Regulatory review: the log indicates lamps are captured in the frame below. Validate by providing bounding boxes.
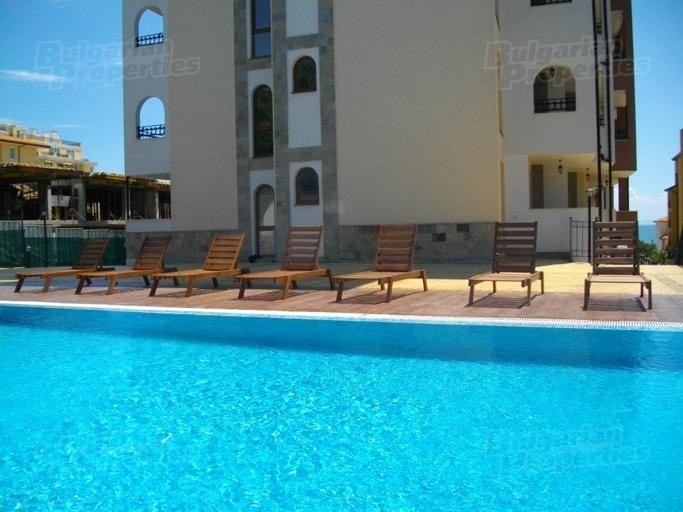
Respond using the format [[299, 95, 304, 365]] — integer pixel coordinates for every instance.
[[557, 160, 609, 187]]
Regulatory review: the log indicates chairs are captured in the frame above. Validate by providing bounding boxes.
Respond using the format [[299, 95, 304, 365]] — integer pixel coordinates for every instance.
[[149, 232, 251, 298], [469, 222, 543, 305], [233, 224, 334, 299], [583, 221, 651, 311], [74, 235, 180, 295], [333, 224, 428, 304], [14, 239, 115, 292]]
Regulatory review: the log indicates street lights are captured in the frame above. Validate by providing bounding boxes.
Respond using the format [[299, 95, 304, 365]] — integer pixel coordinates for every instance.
[[584, 186, 594, 263], [40, 207, 47, 267]]
